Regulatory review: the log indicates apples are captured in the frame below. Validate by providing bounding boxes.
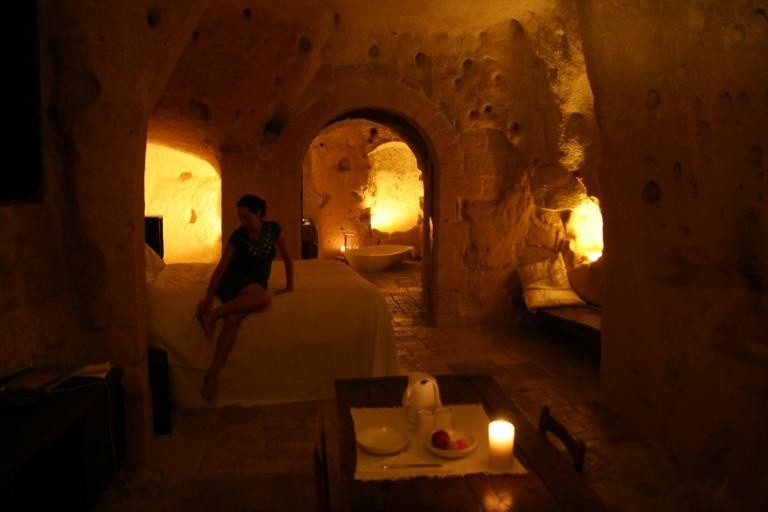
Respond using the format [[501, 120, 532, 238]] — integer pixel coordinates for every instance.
[[431, 430, 450, 449]]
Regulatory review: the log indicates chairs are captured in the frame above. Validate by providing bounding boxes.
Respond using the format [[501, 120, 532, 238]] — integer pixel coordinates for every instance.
[[309, 405, 586, 511]]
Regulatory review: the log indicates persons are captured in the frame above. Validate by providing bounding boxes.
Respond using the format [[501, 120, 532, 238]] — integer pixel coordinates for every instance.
[[194, 193, 294, 401]]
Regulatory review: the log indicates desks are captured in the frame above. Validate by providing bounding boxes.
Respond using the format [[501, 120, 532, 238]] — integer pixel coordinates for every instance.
[[1, 365, 126, 511]]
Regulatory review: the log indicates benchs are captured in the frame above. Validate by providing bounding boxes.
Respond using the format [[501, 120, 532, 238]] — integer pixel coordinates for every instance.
[[512, 287, 601, 342]]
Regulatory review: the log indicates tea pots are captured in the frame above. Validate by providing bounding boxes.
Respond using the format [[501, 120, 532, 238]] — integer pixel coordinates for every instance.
[[403, 377, 440, 422]]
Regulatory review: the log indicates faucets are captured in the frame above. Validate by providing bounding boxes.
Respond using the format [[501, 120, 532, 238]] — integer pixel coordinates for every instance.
[[344, 233, 355, 250]]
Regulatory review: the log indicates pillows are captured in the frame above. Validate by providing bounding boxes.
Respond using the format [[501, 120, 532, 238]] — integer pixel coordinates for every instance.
[[514, 253, 587, 314]]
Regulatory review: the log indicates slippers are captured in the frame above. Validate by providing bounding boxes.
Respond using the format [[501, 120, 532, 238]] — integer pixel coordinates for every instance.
[[202, 372, 217, 400], [197, 313, 214, 340]]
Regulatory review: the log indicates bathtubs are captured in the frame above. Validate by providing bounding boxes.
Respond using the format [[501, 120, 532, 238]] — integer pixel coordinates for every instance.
[[345, 244, 409, 273]]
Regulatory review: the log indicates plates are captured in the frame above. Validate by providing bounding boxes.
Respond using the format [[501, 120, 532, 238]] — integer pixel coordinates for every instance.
[[355, 427, 410, 458], [424, 431, 478, 458]]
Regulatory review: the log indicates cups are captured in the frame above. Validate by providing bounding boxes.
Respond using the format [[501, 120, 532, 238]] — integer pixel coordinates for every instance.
[[416, 407, 451, 437]]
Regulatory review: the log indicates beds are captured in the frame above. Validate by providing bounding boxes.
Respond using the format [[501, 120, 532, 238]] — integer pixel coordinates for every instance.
[[145, 258, 393, 406]]
